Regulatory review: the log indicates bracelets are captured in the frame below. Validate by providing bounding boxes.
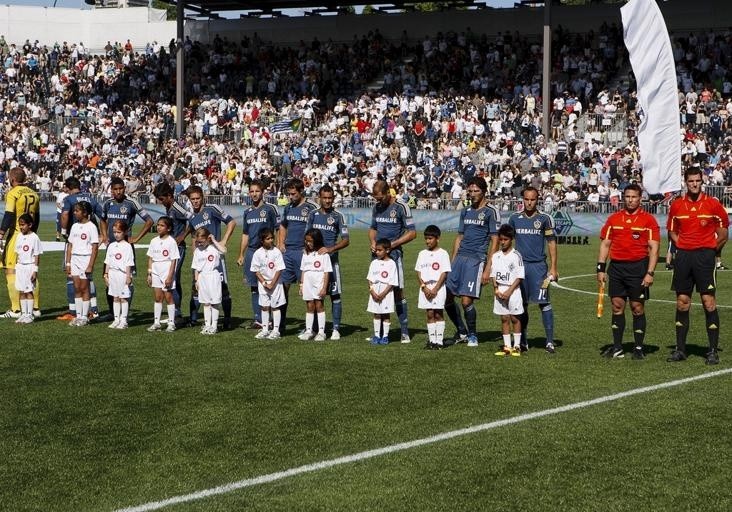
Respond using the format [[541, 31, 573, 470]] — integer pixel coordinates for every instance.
[[493, 285, 498, 289], [368, 285, 373, 289], [369, 287, 374, 291], [103, 275, 107, 278], [262, 281, 268, 287], [146, 272, 152, 277], [194, 279, 198, 285], [298, 281, 304, 284], [421, 283, 425, 288], [422, 286, 426, 290], [494, 289, 500, 294], [298, 283, 303, 287], [261, 281, 266, 286]]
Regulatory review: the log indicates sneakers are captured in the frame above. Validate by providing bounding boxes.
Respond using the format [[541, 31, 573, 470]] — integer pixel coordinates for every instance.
[[547, 342, 557, 352], [665, 264, 673, 270], [0, 309, 41, 323], [365, 333, 388, 344], [186, 317, 231, 334], [496, 345, 529, 357], [245, 322, 281, 339], [299, 328, 342, 341], [400, 332, 411, 344], [600, 347, 624, 357], [632, 345, 644, 359], [57, 311, 100, 325], [424, 333, 479, 350], [705, 348, 718, 365], [146, 316, 184, 332], [109, 318, 129, 329], [717, 263, 728, 269], [667, 349, 689, 361]]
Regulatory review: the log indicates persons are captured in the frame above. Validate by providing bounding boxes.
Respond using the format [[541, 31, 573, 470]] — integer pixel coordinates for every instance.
[[366, 237, 399, 345], [298, 227, 333, 342], [55, 175, 103, 323], [489, 223, 527, 358], [14, 212, 44, 324], [506, 186, 559, 354], [364, 180, 417, 345], [236, 178, 281, 331], [596, 184, 661, 363], [97, 176, 155, 322], [145, 215, 181, 332], [189, 227, 227, 335], [665, 166, 730, 365], [182, 184, 237, 330], [249, 227, 287, 341], [0, 167, 41, 319], [1, 16, 732, 274], [65, 200, 100, 327], [305, 184, 350, 341], [444, 175, 501, 348], [414, 224, 453, 351], [104, 220, 135, 330], [279, 177, 318, 336], [151, 182, 195, 324]]
